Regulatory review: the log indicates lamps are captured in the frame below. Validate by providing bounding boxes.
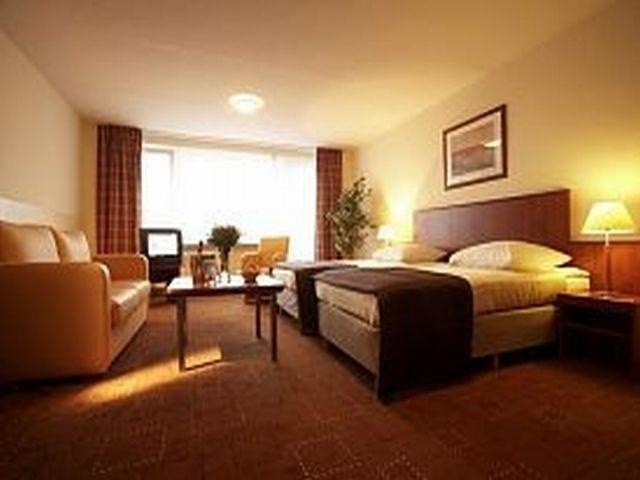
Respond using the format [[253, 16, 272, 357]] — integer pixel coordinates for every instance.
[[376, 223, 397, 247], [228, 93, 263, 113], [581, 201, 635, 293]]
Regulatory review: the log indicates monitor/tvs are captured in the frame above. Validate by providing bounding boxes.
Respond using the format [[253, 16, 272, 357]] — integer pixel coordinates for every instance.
[[146, 232, 179, 260]]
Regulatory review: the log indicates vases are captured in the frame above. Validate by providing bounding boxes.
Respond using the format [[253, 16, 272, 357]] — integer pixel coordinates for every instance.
[[219, 249, 230, 273]]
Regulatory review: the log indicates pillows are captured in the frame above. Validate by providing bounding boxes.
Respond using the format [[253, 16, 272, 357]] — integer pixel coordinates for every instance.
[[375, 242, 447, 265], [449, 238, 572, 273]]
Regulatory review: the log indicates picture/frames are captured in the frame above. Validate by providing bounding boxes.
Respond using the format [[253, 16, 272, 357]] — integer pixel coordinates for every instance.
[[444, 104, 508, 190]]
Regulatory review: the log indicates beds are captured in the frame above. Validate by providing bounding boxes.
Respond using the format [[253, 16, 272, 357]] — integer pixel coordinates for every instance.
[[310, 261, 593, 401], [270, 256, 398, 337]]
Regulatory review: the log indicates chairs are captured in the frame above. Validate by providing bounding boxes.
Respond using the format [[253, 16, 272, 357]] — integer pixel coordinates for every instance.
[[243, 235, 288, 277]]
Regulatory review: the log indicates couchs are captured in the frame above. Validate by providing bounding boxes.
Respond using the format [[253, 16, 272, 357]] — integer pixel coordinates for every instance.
[[0, 222, 151, 386]]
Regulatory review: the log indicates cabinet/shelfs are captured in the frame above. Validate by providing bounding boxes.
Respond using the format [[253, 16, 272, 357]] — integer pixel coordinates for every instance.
[[140, 229, 183, 282]]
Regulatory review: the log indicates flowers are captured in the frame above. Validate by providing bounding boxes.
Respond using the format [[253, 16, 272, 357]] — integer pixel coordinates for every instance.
[[208, 226, 242, 249]]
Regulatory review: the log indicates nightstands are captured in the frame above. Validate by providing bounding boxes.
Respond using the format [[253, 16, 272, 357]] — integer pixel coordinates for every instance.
[[554, 289, 640, 374]]
